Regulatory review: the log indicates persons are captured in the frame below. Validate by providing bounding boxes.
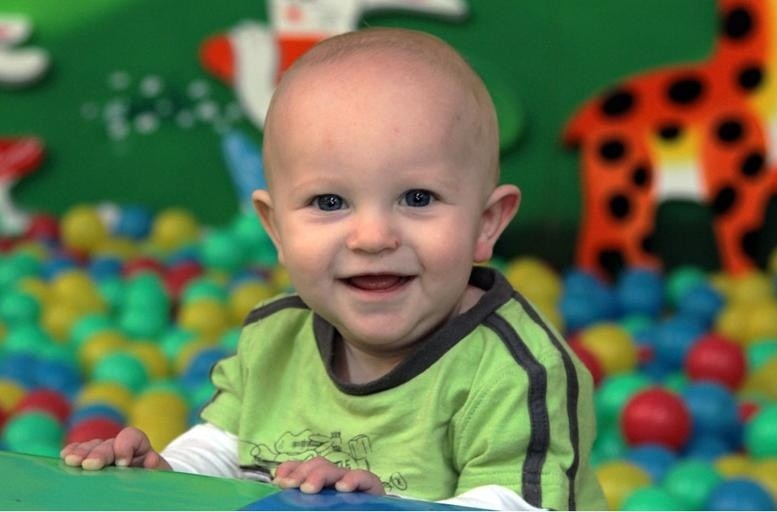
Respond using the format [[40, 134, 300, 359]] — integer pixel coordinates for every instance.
[[59, 24, 612, 512]]
